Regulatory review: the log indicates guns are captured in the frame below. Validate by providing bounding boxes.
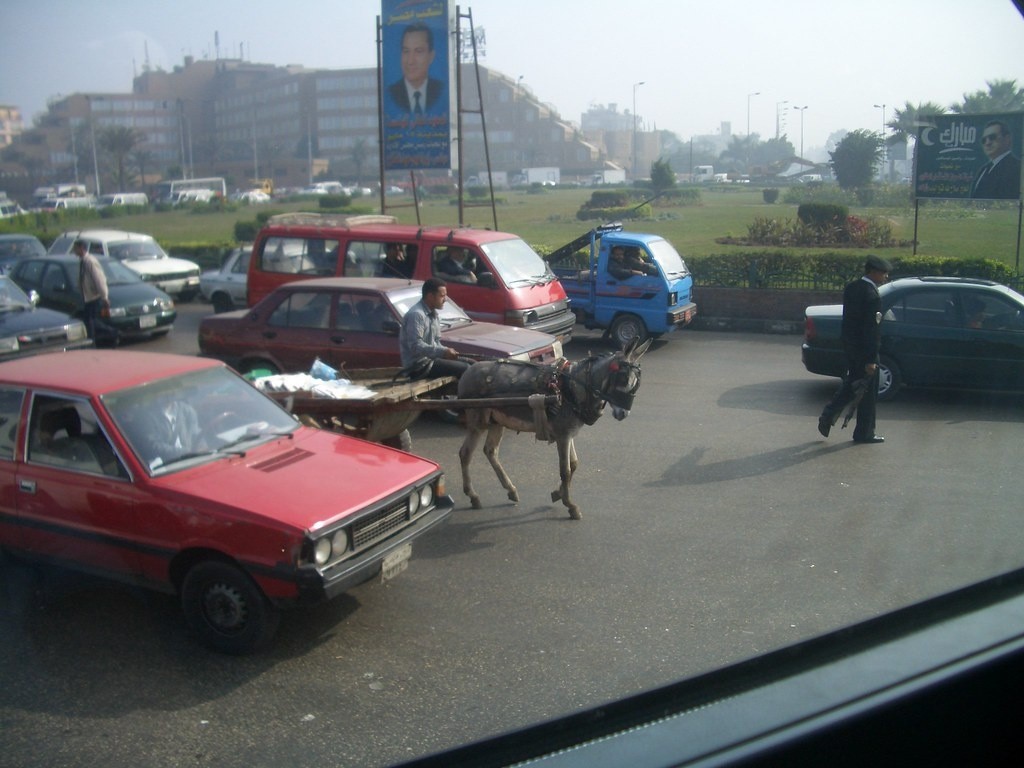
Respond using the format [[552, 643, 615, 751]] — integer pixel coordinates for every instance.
[[841, 363, 879, 430]]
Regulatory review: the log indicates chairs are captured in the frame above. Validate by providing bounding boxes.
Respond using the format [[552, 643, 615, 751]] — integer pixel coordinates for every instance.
[[34, 406, 121, 476], [335, 303, 355, 328], [356, 300, 385, 330]]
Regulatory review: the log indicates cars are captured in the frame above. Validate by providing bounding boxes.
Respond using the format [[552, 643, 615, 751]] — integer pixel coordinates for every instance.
[[0, 347, 458, 655], [198, 242, 334, 314], [0, 272, 95, 361], [674, 166, 821, 184], [0, 232, 48, 274], [45, 228, 202, 303], [197, 275, 567, 425], [800, 275, 1024, 405], [10, 252, 177, 349]]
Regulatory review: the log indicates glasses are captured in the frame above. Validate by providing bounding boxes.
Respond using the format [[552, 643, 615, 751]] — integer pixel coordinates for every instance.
[[981, 133, 996, 144]]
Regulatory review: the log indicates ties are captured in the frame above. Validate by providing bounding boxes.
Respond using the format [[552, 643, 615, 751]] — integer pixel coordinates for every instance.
[[982, 162, 994, 177], [412, 92, 422, 115]]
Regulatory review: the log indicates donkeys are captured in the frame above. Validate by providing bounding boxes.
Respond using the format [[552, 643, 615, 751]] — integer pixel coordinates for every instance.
[[456, 335, 653, 518]]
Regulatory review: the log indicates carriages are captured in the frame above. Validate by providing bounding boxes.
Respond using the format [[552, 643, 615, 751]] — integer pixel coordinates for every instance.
[[217, 332, 656, 522]]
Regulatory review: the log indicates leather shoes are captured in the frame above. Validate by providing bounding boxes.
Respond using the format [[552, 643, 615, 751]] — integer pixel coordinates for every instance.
[[854, 436, 884, 442], [818, 415, 831, 437]]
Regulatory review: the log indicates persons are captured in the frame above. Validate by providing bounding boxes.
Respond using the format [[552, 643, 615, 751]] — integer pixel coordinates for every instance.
[[75, 240, 122, 347], [376, 242, 418, 279], [398, 278, 478, 425], [959, 294, 1013, 330], [130, 388, 206, 463], [607, 246, 657, 280], [388, 22, 443, 114], [439, 245, 503, 286], [818, 253, 892, 441]]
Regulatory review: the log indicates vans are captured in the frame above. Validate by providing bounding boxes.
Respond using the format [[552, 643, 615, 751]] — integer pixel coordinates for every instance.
[[247, 212, 576, 346]]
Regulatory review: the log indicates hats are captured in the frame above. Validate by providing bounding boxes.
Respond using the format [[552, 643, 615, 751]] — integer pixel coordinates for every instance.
[[867, 254, 893, 273]]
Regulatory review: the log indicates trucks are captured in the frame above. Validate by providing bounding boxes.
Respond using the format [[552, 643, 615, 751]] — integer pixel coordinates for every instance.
[[543, 223, 699, 350], [0, 167, 626, 220]]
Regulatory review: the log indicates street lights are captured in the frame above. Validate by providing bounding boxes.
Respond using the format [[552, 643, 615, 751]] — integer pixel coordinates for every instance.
[[516, 74, 525, 172], [747, 92, 761, 165], [873, 103, 887, 186], [633, 81, 646, 180], [793, 105, 808, 171]]
